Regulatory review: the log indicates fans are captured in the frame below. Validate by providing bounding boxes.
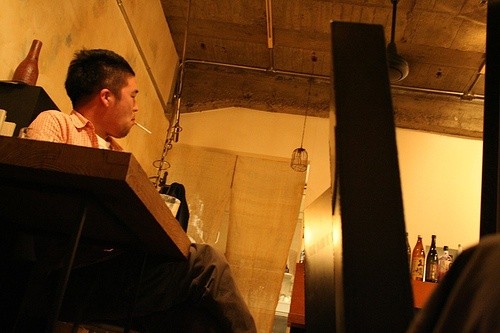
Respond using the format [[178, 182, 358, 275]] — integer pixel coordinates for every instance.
[[386, 0, 409, 83]]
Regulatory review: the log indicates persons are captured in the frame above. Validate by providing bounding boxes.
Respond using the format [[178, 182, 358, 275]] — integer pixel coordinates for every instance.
[[24, 48, 257, 333]]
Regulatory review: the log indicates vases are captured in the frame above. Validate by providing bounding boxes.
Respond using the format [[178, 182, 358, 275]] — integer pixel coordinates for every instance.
[[13, 40, 43, 85]]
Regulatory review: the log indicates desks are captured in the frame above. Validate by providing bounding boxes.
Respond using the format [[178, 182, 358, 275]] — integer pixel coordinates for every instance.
[[288, 253, 308, 325], [0, 134, 190, 333]]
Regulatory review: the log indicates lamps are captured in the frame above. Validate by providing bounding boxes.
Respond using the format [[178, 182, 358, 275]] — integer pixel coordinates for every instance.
[[291, 56, 318, 171]]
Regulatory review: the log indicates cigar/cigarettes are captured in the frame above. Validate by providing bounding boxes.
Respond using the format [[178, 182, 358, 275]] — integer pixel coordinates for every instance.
[[134, 121, 152, 135]]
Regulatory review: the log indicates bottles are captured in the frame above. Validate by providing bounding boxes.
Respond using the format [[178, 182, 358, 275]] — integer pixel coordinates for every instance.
[[12, 39, 42, 86], [457, 243, 462, 254], [405, 232, 411, 267], [426, 235, 438, 282], [438, 246, 452, 282], [412, 234, 426, 282]]
